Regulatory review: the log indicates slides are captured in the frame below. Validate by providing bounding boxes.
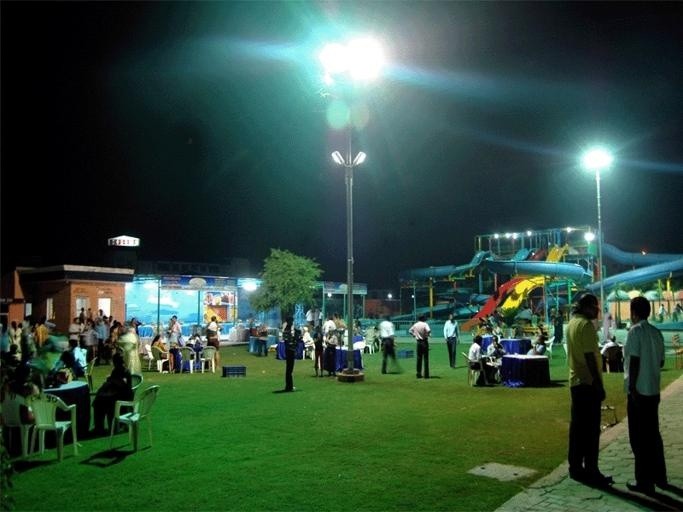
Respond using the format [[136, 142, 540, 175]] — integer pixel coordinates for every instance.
[[459, 246, 566, 332]]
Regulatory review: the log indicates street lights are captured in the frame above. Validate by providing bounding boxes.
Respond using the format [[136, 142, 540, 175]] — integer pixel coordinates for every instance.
[[318, 34, 389, 382], [578, 142, 617, 345]]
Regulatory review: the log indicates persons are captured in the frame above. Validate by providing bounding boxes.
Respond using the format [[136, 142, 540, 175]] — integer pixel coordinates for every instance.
[[468, 334, 493, 385], [88, 352, 134, 435], [274, 305, 381, 377], [407, 314, 432, 379], [565, 291, 614, 483], [378, 314, 399, 375], [258, 323, 268, 357], [622, 294, 672, 496], [283, 317, 301, 393], [443, 313, 461, 369], [487, 335, 506, 355], [658, 304, 683, 322], [8, 364, 41, 424], [600, 307, 620, 357], [526, 334, 545, 355], [0, 305, 239, 375], [554, 311, 564, 344]]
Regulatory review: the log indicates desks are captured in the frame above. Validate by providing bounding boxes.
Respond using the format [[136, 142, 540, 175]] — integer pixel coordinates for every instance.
[[475, 333, 503, 352], [501, 352, 551, 390], [0, 380, 98, 445], [498, 337, 534, 355]]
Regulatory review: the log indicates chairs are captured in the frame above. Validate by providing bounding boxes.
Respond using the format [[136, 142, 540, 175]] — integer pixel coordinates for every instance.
[[670, 331, 682, 374], [604, 346, 626, 374], [461, 350, 494, 388], [541, 346, 547, 356], [596, 400, 618, 426], [542, 336, 556, 355], [25, 390, 82, 462], [107, 384, 160, 455], [0, 386, 37, 462]]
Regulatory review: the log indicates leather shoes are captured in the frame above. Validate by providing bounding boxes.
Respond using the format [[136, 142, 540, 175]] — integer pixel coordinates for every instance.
[[588, 474, 612, 484], [627, 479, 654, 492]]
